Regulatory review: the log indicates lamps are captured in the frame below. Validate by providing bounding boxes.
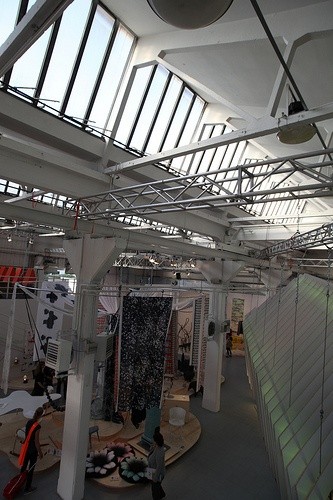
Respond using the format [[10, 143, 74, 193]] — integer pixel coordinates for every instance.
[[277, 99, 316, 145], [145, 0, 234, 31]]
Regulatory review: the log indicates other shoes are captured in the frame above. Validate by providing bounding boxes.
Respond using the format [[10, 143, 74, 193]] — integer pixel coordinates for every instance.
[[24, 487, 37, 496], [230, 355, 232, 357], [226, 356, 229, 358]]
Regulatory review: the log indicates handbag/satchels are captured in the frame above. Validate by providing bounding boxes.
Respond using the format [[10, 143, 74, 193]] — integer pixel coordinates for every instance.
[[18, 444, 28, 466]]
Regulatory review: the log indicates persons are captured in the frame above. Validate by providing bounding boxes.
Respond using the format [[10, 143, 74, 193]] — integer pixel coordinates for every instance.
[[20, 407, 43, 491], [146, 432, 166, 500], [31, 360, 48, 396], [226, 333, 232, 358]]
